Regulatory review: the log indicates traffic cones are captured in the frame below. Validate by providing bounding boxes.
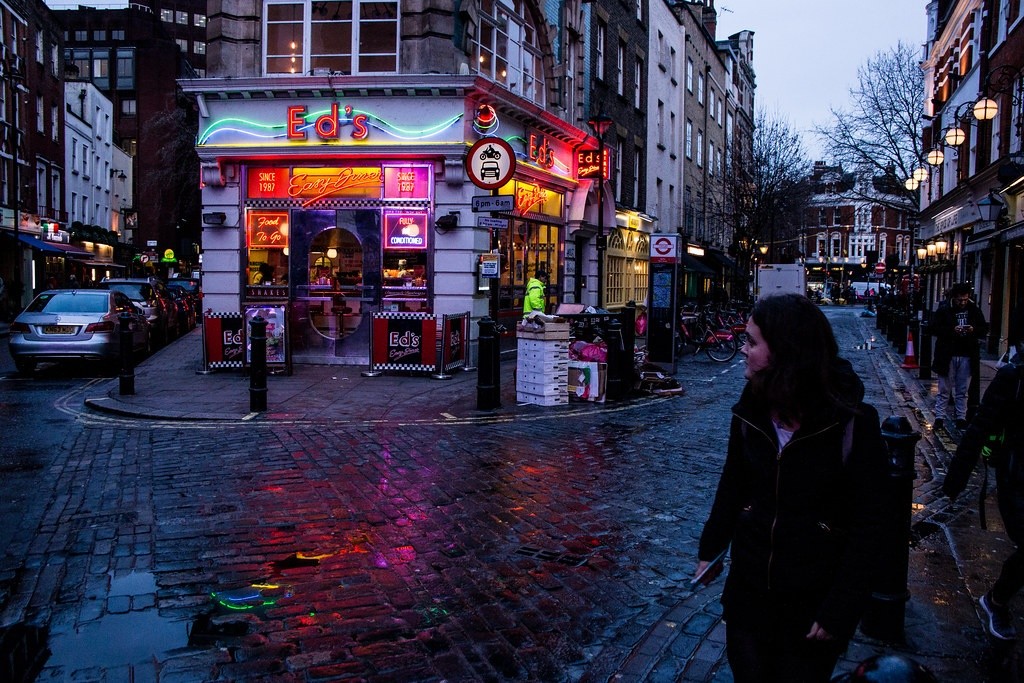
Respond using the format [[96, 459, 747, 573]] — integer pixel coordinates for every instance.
[[901, 331, 922, 369]]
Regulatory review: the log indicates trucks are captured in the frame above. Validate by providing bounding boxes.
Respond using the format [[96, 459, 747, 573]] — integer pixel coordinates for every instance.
[[757, 264, 808, 301]]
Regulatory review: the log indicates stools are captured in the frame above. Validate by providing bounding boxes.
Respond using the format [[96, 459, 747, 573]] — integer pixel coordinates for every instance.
[[308, 305, 324, 335], [331, 305, 353, 338]]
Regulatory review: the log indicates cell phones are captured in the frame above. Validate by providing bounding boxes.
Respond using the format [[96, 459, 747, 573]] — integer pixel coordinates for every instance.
[[963, 325, 970, 330], [689, 549, 729, 592]]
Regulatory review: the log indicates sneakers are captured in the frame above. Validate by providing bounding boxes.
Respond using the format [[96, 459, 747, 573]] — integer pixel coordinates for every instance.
[[979, 595, 1018, 640]]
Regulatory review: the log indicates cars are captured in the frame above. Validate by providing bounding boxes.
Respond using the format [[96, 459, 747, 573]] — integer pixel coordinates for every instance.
[[160, 284, 198, 333], [9, 289, 153, 376]]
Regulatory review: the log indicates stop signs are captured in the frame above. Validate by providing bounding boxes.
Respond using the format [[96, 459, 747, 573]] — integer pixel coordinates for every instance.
[[876, 262, 886, 274]]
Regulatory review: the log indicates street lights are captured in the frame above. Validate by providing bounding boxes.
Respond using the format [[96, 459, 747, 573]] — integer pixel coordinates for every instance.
[[588, 110, 610, 308], [754, 241, 768, 300], [860, 260, 870, 292], [907, 216, 917, 271]]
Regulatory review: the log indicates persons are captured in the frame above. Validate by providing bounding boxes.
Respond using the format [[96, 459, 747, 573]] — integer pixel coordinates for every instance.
[[695, 292, 888, 683], [522, 271, 547, 318], [941, 350, 1024, 642], [927, 282, 988, 430]]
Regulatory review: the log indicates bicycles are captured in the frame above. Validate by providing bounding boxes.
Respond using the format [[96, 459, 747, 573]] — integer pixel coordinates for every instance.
[[676, 296, 749, 362]]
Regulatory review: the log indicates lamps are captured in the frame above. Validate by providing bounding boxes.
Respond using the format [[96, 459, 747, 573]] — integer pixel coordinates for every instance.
[[435, 211, 460, 231], [110, 168, 127, 182], [946, 101, 978, 147], [203, 212, 226, 226], [926, 127, 958, 167], [972, 65, 1023, 137], [905, 151, 944, 190], [917, 234, 948, 265], [977, 187, 1004, 221], [472, 265, 490, 290]]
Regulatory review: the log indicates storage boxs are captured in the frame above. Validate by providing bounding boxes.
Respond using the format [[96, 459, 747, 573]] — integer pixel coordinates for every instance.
[[517, 320, 570, 406]]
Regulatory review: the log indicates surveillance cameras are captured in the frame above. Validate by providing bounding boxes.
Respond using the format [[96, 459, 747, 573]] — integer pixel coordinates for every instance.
[[196, 95, 210, 118]]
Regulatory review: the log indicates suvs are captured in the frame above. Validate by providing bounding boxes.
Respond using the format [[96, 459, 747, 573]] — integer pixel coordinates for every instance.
[[166, 278, 202, 323], [99, 276, 180, 345]]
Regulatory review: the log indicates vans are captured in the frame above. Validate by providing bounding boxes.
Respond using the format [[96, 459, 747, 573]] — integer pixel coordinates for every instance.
[[801, 262, 898, 298]]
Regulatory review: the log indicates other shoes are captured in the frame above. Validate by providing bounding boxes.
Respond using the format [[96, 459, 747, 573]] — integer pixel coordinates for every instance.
[[958, 418, 968, 429], [933, 418, 944, 430]]
